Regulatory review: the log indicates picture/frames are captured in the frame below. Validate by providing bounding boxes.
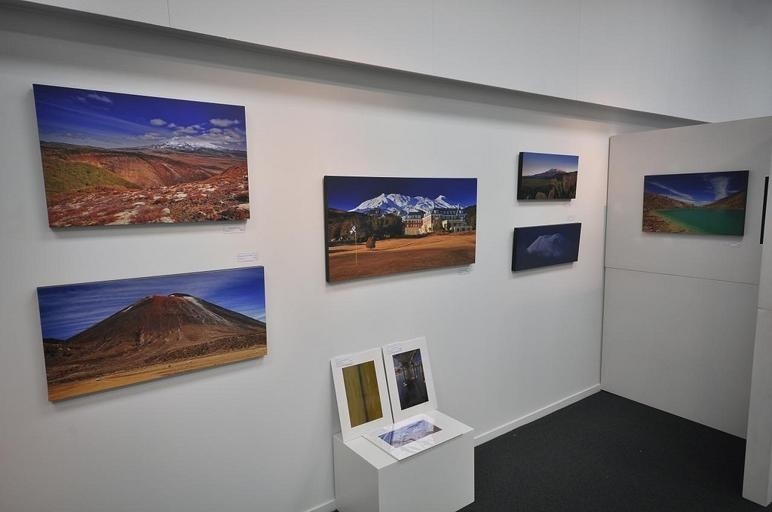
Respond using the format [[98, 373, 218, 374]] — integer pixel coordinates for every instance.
[[382, 334, 439, 425], [361, 413, 462, 461], [330, 347, 394, 442]]
